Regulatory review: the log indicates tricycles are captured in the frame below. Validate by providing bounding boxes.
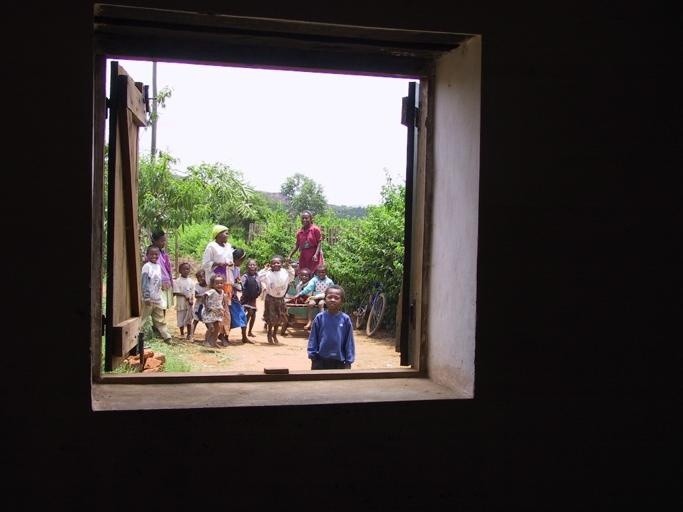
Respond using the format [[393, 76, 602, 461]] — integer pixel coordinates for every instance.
[[278, 301, 339, 334]]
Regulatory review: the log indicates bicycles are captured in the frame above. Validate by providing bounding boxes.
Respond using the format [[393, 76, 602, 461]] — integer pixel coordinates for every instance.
[[349, 256, 396, 337]]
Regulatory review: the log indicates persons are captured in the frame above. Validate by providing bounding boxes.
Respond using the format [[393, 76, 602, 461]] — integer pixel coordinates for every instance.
[[307, 285, 355, 369], [140, 210, 334, 349]]
